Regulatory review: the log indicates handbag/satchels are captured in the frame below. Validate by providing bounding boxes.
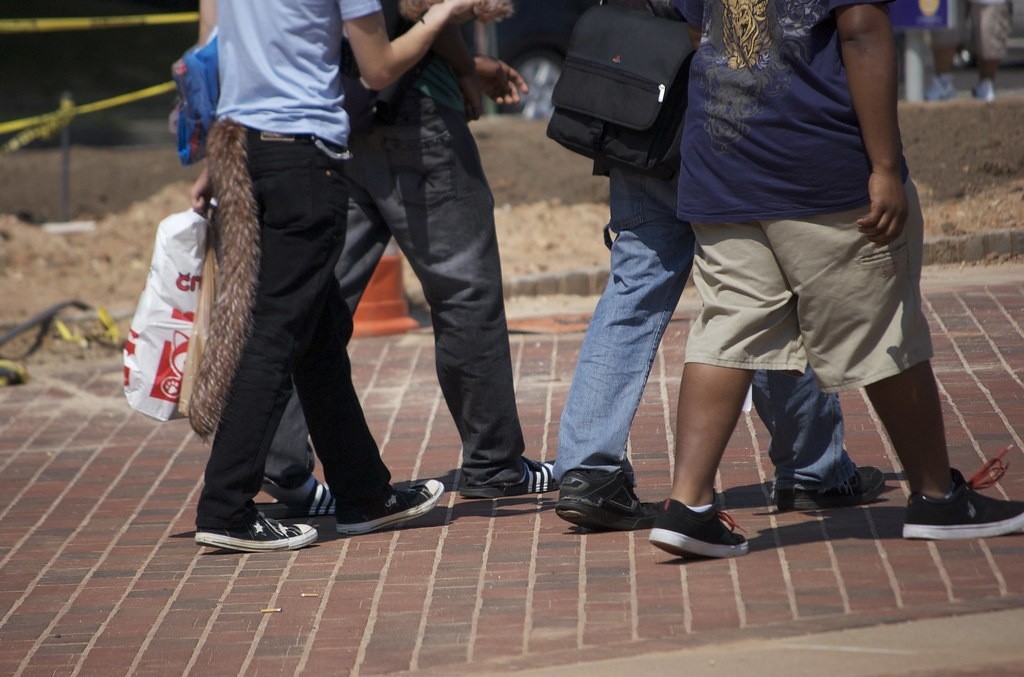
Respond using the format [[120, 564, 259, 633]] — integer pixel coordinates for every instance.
[[173, 28, 220, 168], [544, 8, 696, 180], [122, 207, 207, 422]]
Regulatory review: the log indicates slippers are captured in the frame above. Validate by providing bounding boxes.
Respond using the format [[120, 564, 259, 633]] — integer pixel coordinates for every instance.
[[253, 479, 335, 519], [460, 456, 560, 498]]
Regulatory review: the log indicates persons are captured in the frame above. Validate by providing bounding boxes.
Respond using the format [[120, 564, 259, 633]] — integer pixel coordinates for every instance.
[[546, 0, 1024, 558], [169, 0, 557, 550]]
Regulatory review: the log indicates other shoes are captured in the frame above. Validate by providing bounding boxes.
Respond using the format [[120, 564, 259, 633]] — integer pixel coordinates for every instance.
[[926, 75, 955, 101], [972, 78, 994, 101]]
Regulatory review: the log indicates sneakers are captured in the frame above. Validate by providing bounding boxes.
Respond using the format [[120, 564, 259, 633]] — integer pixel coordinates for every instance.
[[193, 512, 318, 553], [334, 478, 446, 534], [556, 468, 660, 530], [775, 467, 884, 511], [648, 489, 748, 557], [902, 444, 1024, 539]]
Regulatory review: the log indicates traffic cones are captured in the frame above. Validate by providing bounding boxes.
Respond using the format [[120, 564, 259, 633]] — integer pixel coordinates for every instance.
[[350, 237, 418, 337]]
